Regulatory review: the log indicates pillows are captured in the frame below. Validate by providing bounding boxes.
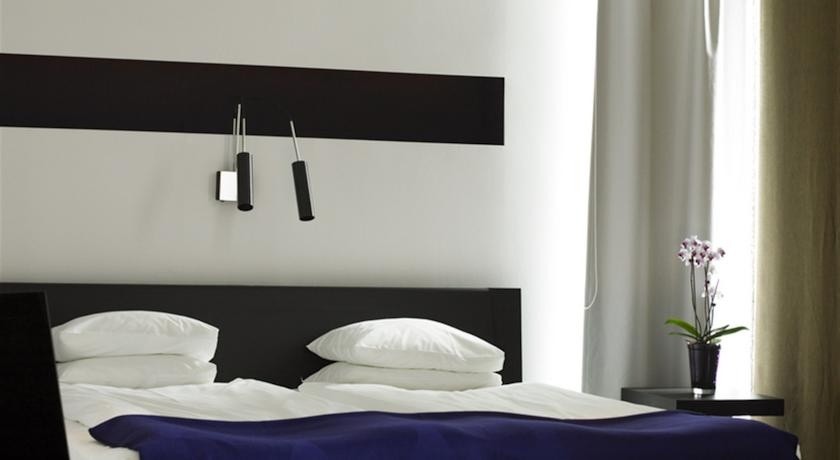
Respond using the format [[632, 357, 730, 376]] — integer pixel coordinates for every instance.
[[305, 318, 505, 373], [53, 353, 218, 390], [49, 310, 220, 365], [297, 362, 502, 393]]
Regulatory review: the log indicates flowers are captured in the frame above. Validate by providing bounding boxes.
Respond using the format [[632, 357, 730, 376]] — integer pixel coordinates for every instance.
[[662, 235, 749, 344]]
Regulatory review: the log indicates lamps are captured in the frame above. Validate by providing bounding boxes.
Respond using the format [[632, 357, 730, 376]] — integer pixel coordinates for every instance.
[[290, 120, 315, 222], [215, 103, 242, 203], [237, 118, 255, 212]]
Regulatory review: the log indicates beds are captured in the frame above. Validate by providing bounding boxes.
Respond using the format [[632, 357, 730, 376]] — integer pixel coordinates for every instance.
[[56, 376, 805, 460]]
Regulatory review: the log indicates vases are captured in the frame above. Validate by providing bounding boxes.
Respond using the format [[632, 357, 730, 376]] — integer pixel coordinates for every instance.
[[687, 341, 721, 395]]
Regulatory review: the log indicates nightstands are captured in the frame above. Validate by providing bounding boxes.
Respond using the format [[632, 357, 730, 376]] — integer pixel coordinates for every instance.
[[621, 386, 785, 419]]
